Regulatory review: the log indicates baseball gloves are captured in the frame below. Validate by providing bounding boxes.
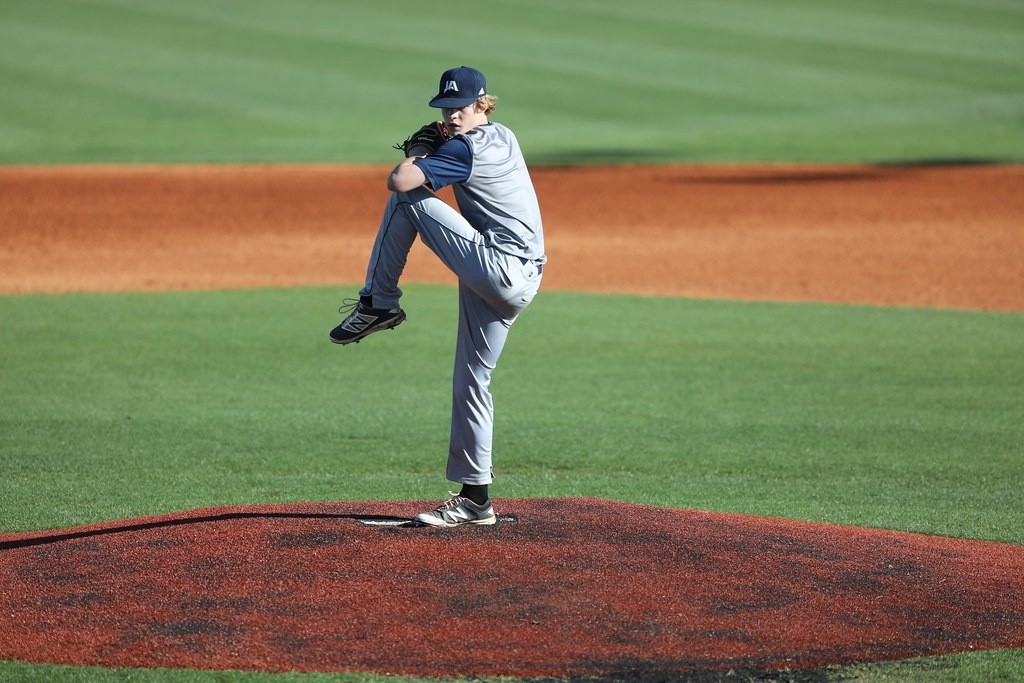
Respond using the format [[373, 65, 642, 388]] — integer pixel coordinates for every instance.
[[405, 119, 450, 155]]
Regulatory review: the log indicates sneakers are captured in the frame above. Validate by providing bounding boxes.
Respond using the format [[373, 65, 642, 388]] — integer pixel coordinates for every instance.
[[414, 496, 497, 528], [328, 300, 406, 345]]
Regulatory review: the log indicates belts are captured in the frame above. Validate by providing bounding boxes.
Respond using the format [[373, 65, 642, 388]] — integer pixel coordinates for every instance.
[[517, 256, 542, 275]]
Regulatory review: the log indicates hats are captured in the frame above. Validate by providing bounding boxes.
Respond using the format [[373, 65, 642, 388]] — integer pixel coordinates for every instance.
[[429, 66, 487, 109]]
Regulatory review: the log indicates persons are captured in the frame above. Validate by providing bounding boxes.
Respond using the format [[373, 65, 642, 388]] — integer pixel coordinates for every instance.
[[329, 66, 548, 529]]
